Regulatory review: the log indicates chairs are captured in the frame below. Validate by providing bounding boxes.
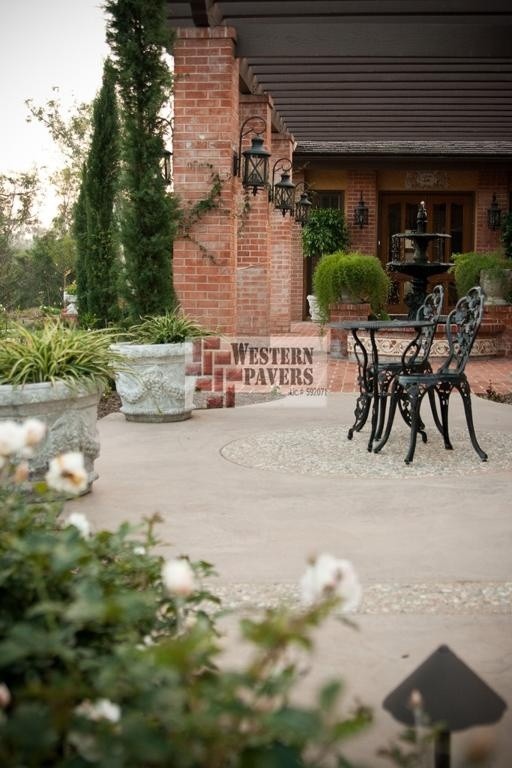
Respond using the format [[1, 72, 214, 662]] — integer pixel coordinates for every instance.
[[345, 282, 488, 468]]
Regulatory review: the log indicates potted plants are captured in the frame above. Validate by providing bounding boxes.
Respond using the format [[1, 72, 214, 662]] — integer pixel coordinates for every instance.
[[445, 245, 510, 319], [109, 308, 218, 422], [310, 247, 393, 354], [2, 306, 134, 505]]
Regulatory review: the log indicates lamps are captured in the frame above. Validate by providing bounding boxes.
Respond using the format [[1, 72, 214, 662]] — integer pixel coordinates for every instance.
[[486, 190, 501, 232], [231, 114, 312, 230], [351, 187, 369, 229]]
